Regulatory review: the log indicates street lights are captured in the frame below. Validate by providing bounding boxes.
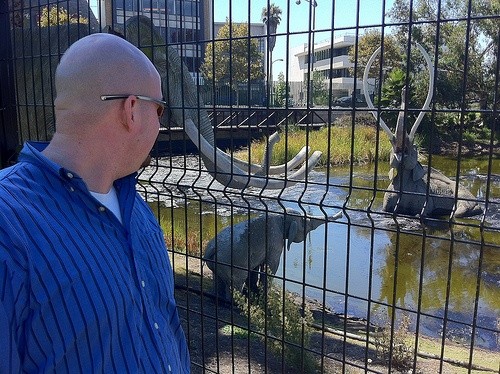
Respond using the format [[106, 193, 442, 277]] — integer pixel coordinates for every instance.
[[295, 0, 319, 109], [270, 58, 283, 106]]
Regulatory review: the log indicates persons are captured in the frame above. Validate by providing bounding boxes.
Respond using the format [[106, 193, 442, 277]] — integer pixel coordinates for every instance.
[[1, 33, 193, 374]]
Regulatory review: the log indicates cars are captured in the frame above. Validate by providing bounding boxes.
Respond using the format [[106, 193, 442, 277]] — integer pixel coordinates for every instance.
[[336, 96, 368, 108]]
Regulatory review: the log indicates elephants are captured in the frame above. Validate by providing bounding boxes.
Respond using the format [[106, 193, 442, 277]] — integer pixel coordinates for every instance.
[[199, 208, 342, 311], [362, 42, 484, 223], [1, 0, 323, 190]]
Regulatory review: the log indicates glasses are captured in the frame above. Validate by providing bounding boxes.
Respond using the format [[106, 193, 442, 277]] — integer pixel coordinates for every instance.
[[100, 94, 167, 117]]
